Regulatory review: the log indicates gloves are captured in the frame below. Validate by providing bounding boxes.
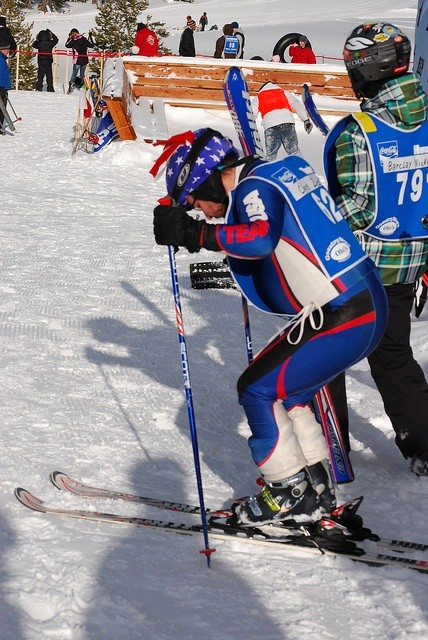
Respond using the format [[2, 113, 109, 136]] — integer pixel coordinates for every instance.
[[152, 203, 220, 253], [413, 272, 428, 319], [304, 120, 313, 135]]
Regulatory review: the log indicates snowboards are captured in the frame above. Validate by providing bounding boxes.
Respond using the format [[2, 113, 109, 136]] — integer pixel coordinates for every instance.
[[223, 67, 353, 484], [90, 112, 119, 152], [301, 82, 330, 137]]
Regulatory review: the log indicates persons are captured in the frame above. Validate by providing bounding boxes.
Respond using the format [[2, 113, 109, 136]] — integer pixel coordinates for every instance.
[[251, 81, 313, 161], [32, 29, 59, 92], [134, 23, 158, 56], [0, 13, 18, 76], [199, 12, 208, 31], [310, 22, 428, 478], [214, 24, 240, 59], [0, 37, 13, 135], [65, 28, 94, 94], [143, 127, 389, 528], [179, 16, 196, 57], [231, 22, 245, 59], [289, 36, 316, 64]]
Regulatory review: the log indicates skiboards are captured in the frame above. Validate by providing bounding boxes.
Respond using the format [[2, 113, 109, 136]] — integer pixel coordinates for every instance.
[[14, 472, 428, 574]]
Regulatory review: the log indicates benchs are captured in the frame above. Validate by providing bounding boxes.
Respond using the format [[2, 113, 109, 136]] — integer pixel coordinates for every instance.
[[125, 58, 361, 115], [103, 61, 169, 146]]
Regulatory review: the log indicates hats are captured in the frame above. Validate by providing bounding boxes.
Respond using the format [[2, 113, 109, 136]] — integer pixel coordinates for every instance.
[[136, 23, 146, 31], [0, 40, 11, 49], [231, 21, 239, 28], [186, 16, 195, 29], [299, 35, 307, 41]]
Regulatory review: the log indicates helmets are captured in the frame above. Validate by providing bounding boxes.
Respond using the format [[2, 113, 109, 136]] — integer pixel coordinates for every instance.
[[144, 127, 239, 205], [342, 20, 411, 99]]
